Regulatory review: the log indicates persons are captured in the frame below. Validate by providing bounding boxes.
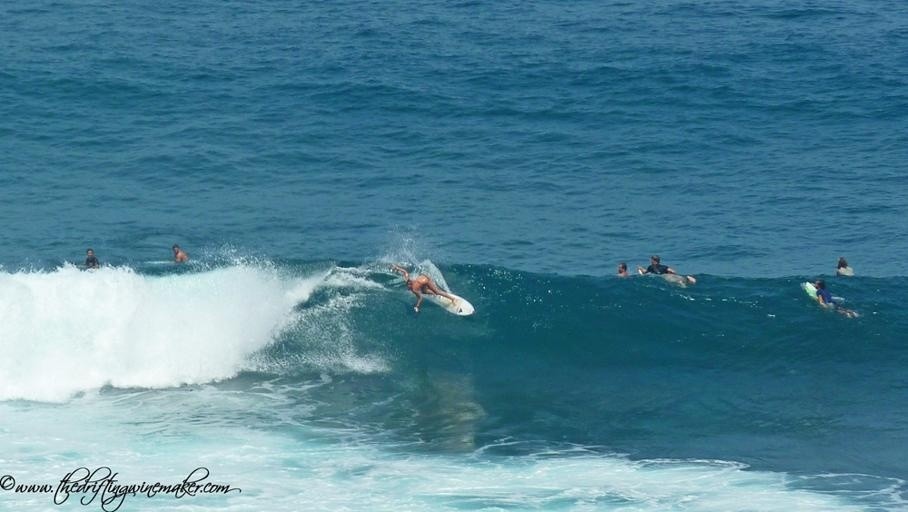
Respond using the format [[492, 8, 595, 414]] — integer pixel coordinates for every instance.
[[639, 255, 674, 275], [173, 243, 188, 262], [85, 248, 100, 268], [616, 263, 630, 277], [836, 256, 847, 268], [391, 263, 455, 313], [814, 278, 857, 318]]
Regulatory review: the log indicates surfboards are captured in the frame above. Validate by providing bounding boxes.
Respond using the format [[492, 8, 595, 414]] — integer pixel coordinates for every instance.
[[806, 282, 818, 299], [429, 293, 475, 316]]
